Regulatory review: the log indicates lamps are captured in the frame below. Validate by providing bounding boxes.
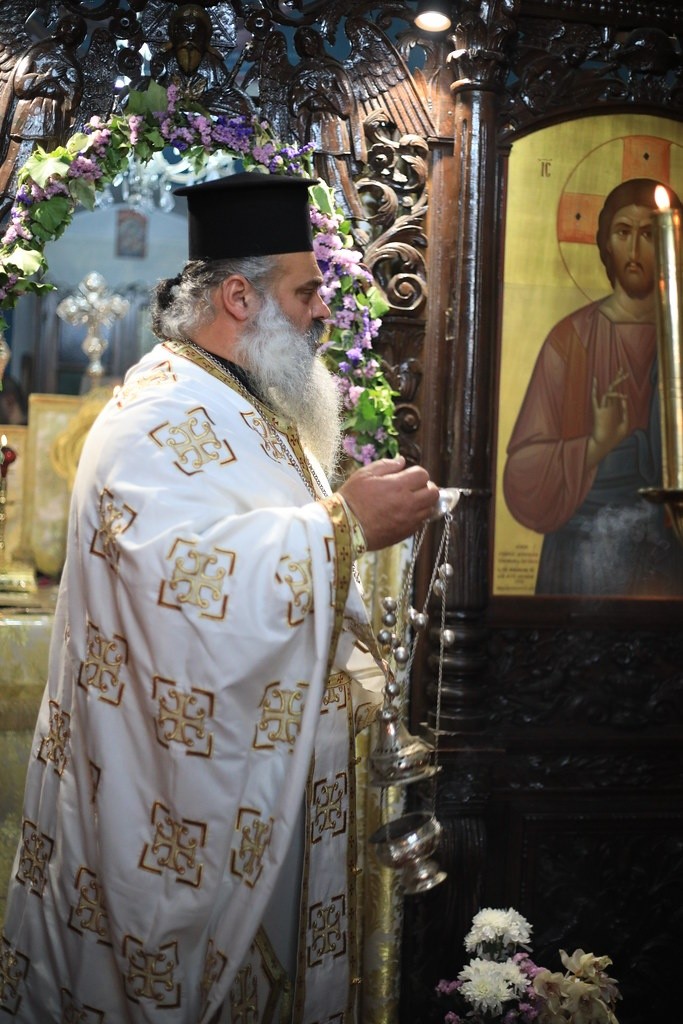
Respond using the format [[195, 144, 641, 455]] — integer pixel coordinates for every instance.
[[414, 0, 452, 32]]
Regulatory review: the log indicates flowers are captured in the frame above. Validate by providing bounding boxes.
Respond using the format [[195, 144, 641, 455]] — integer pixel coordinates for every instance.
[[1, 84, 400, 468], [435, 904, 622, 1024]]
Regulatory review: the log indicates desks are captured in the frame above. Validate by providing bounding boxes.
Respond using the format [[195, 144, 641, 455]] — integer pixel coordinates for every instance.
[[0, 601, 405, 1024]]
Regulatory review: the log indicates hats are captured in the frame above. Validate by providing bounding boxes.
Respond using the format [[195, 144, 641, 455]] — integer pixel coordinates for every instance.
[[172, 171, 321, 261]]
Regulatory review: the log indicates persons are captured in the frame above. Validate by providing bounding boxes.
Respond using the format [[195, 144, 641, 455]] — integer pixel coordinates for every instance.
[[0, 173, 439, 1024]]
